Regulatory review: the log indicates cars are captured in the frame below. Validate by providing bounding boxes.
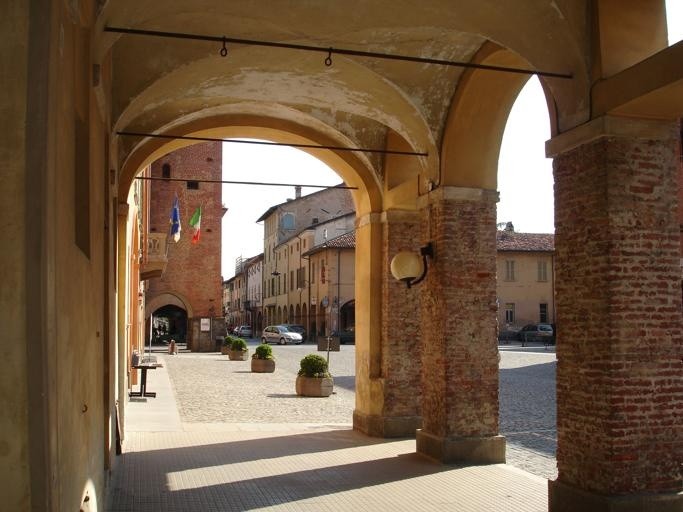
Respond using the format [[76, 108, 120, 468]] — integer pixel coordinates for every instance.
[[234, 326, 253, 338], [500, 324, 553, 342], [262, 325, 307, 345]]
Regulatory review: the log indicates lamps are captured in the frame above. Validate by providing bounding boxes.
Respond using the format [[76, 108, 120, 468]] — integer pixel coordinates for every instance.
[[390, 241, 434, 290]]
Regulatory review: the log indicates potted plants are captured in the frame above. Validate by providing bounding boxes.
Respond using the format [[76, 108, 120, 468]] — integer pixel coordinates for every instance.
[[251, 343, 275, 373], [221, 334, 234, 354], [229, 337, 249, 361], [295, 354, 334, 397]]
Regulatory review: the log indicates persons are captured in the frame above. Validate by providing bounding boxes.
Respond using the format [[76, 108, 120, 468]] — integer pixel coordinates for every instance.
[[162, 325, 166, 335], [158, 324, 161, 335]]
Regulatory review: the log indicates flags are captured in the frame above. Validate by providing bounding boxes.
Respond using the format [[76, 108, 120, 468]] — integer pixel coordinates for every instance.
[[169, 191, 181, 243], [188, 206, 202, 245]]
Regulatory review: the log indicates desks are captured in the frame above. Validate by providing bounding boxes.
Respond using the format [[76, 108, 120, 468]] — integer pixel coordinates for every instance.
[[129, 362, 157, 397]]
